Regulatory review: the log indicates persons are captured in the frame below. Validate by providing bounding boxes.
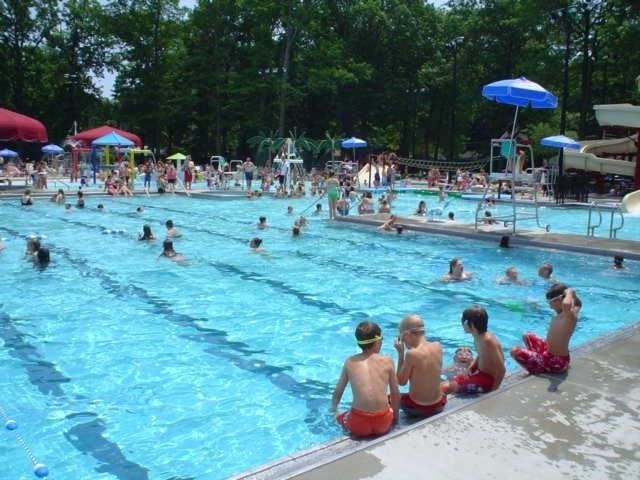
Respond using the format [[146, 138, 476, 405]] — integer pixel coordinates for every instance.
[[484, 211, 499, 225], [377, 214, 397, 231], [312, 174, 327, 198], [138, 224, 156, 240], [290, 228, 301, 238], [339, 175, 352, 186], [157, 239, 187, 264], [183, 155, 200, 190], [292, 216, 310, 228], [164, 220, 183, 239], [441, 306, 506, 398], [21, 189, 33, 205], [358, 191, 374, 213], [427, 168, 556, 194], [275, 155, 289, 187], [497, 122, 525, 173], [26, 239, 41, 257], [378, 191, 392, 213], [274, 187, 286, 198], [448, 212, 454, 220], [387, 314, 447, 419], [326, 171, 340, 221], [207, 174, 217, 191], [157, 160, 178, 193], [246, 189, 262, 199], [240, 157, 257, 190], [440, 257, 471, 282], [607, 256, 630, 272], [64, 203, 72, 212], [75, 191, 85, 209], [349, 187, 361, 201], [510, 284, 582, 375], [337, 194, 350, 216], [257, 216, 269, 230], [416, 201, 427, 216], [496, 267, 527, 286], [263, 168, 271, 192], [315, 203, 324, 215], [329, 321, 400, 440], [443, 347, 474, 373], [485, 198, 496, 207], [288, 182, 306, 198], [104, 171, 133, 197], [498, 236, 514, 248], [136, 206, 145, 216], [139, 163, 153, 194], [0, 150, 54, 188], [56, 189, 64, 202], [33, 248, 50, 272], [249, 237, 269, 257], [539, 262, 553, 279], [345, 181, 352, 197], [373, 172, 381, 187], [287, 207, 293, 214]]
